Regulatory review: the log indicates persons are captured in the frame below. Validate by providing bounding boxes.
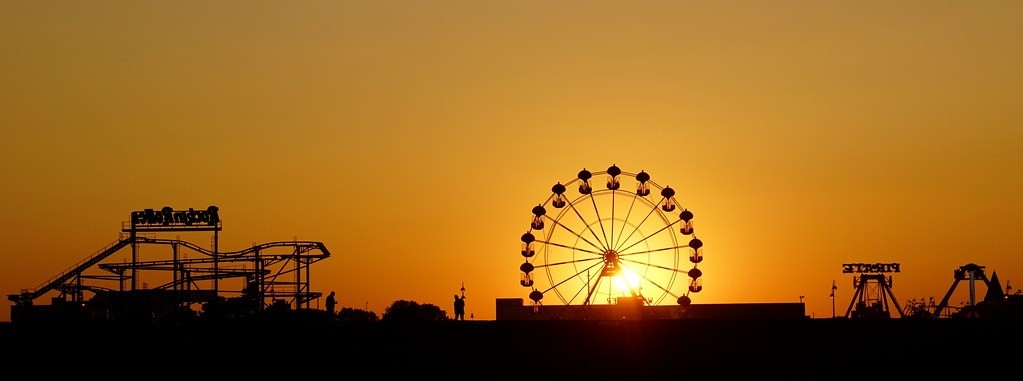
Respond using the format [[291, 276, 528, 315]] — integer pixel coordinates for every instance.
[[325, 291, 338, 312], [454, 295, 465, 320]]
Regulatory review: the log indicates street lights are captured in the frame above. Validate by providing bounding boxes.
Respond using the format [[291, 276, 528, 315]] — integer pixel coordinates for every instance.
[[830, 279, 837, 317], [799, 295, 805, 303], [1006, 279, 1012, 295]]
